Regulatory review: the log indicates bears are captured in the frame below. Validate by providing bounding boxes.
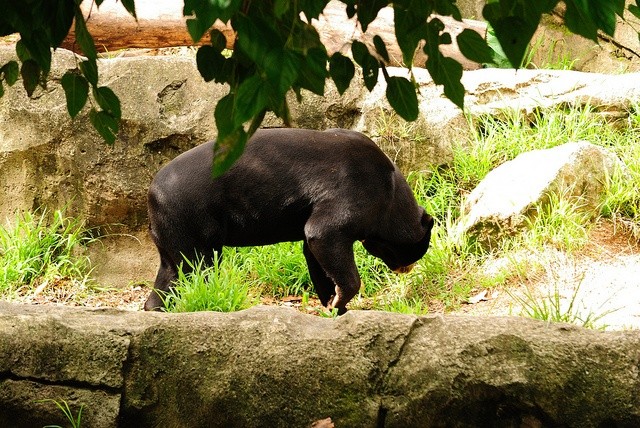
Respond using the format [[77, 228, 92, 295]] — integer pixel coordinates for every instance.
[[143, 126, 436, 316]]
